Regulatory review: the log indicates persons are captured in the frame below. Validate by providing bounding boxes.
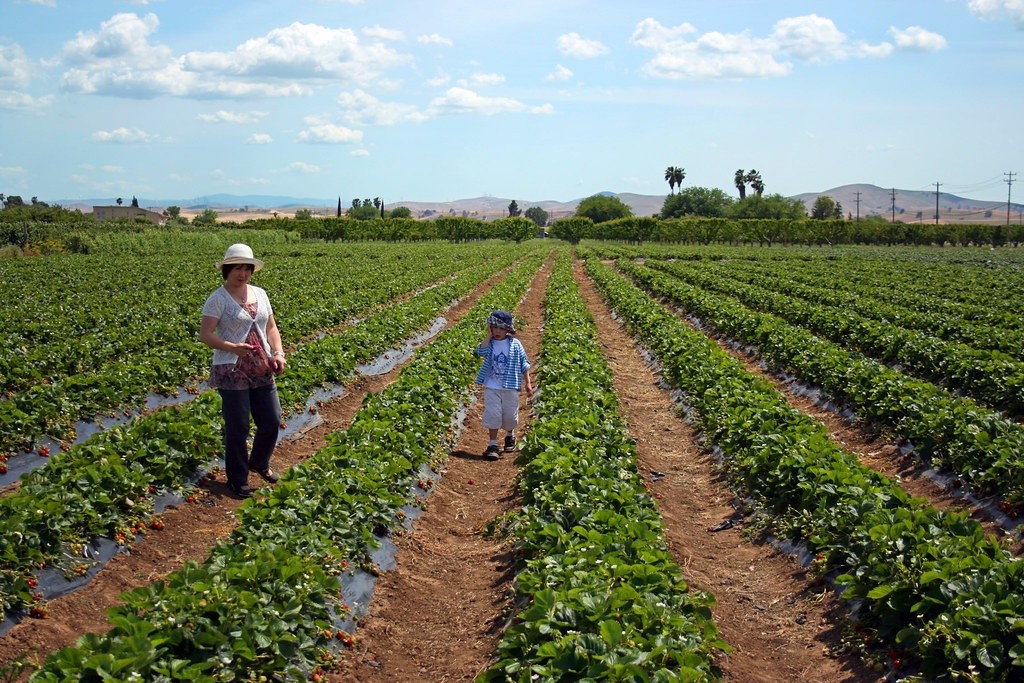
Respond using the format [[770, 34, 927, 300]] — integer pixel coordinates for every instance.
[[475, 312, 532, 460], [199, 244, 287, 498]]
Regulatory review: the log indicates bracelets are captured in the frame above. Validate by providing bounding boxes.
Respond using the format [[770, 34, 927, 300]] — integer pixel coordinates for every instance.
[[273, 351, 285, 357]]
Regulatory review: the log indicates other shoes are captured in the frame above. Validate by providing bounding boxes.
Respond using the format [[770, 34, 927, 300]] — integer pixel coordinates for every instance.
[[225, 479, 254, 497], [251, 468, 278, 483]]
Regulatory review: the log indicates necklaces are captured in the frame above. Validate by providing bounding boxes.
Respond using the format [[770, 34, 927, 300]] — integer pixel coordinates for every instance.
[[228, 284, 246, 303]]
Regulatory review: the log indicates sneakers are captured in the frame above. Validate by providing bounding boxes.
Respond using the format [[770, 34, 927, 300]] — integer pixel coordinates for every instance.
[[504, 436, 516, 452], [483, 446, 499, 460]]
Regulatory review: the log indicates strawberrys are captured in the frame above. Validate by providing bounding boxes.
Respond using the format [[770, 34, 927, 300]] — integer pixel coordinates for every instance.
[[252, 345, 260, 350], [0, 375, 206, 475], [310, 476, 434, 683], [28, 403, 317, 600]]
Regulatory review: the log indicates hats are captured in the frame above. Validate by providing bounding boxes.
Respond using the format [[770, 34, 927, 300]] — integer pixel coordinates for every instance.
[[487, 310, 516, 335], [215, 243, 263, 272]]
